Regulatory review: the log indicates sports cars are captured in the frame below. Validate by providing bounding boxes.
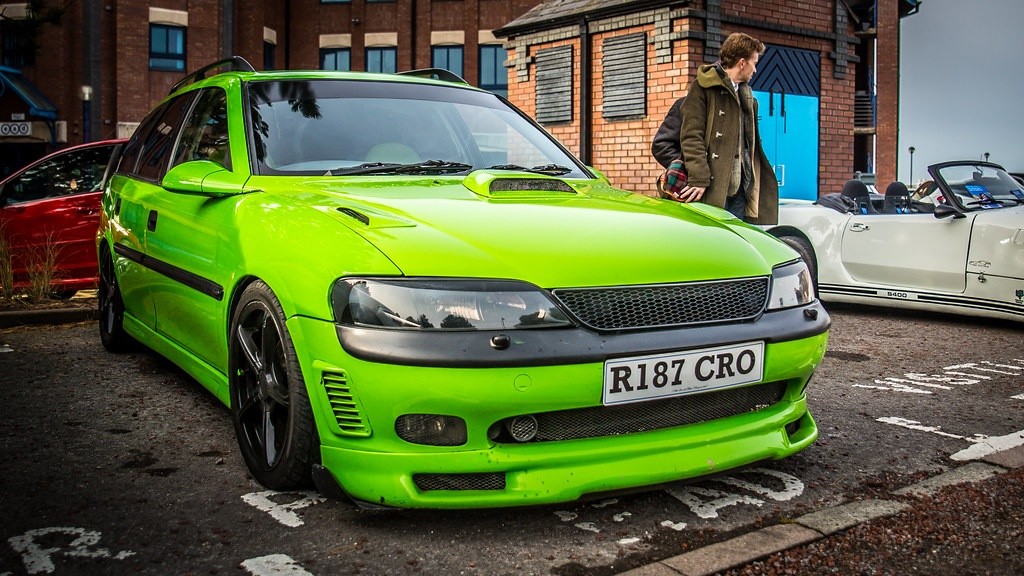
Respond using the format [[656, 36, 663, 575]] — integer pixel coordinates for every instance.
[[745, 159, 1024, 322]]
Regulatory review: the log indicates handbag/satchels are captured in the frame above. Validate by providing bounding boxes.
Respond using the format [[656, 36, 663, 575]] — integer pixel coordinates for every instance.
[[655, 160, 690, 202]]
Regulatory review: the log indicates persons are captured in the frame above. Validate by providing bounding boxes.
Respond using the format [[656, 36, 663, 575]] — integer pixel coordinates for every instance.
[[980, 193, 989, 199], [679, 32, 780, 227], [652, 96, 688, 168]]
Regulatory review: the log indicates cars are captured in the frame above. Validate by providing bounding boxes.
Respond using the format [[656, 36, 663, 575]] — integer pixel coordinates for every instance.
[[0, 136, 183, 302], [94, 56, 834, 510]]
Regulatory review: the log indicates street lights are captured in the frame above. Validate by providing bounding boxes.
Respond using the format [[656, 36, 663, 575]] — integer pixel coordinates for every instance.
[[908, 147, 916, 189], [984, 152, 990, 162]]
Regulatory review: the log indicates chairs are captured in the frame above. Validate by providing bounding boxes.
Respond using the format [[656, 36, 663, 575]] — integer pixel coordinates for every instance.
[[366, 142, 422, 164], [884, 181, 915, 214], [842, 178, 880, 216]]
